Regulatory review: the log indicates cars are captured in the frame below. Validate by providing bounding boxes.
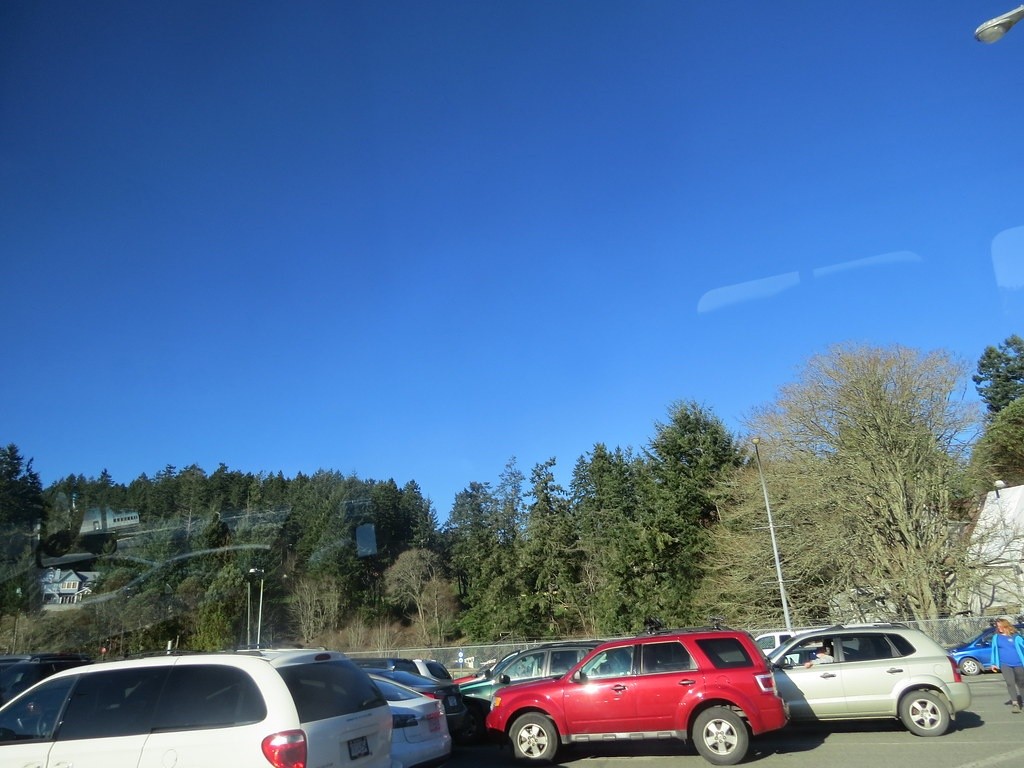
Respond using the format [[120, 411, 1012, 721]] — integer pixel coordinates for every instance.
[[766, 621, 972, 737], [950, 621, 1024, 676], [206, 671, 453, 768], [361, 668, 468, 730], [454, 661, 498, 686]]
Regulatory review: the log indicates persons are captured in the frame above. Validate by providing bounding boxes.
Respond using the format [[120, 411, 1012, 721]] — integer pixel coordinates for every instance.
[[804, 649, 834, 668], [990, 619, 1024, 713]]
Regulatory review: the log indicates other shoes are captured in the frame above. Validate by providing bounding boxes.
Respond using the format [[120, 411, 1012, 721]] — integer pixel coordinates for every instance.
[[1012, 702, 1020, 713]]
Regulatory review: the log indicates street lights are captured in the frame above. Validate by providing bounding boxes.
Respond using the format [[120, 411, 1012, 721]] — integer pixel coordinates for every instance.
[[752, 435, 792, 630], [993, 480, 1023, 604], [247, 568, 266, 649]]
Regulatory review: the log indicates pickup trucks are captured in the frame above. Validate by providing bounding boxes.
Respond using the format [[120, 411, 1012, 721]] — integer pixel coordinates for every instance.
[[751, 621, 893, 656]]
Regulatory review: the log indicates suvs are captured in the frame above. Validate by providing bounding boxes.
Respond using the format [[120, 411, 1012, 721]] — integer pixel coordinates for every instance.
[[349, 656, 453, 684], [0, 655, 95, 718], [485, 624, 791, 768], [0, 648, 407, 768], [459, 640, 633, 740]]
